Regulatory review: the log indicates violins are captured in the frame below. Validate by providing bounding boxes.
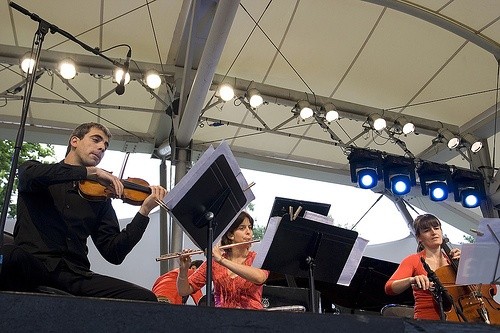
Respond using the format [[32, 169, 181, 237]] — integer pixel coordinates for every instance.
[[73, 168, 152, 206]]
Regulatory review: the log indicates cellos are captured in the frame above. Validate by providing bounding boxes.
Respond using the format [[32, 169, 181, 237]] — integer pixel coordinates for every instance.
[[434, 235, 500, 325]]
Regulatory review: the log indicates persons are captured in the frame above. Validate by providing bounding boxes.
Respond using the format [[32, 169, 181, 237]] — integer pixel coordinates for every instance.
[[151, 258, 205, 305], [385, 213, 471, 321], [3, 121, 169, 303], [177, 210, 269, 309]]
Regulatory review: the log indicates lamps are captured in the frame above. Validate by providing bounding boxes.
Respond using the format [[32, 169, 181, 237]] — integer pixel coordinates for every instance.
[[419, 160, 449, 202], [20, 53, 37, 74], [146, 69, 160, 89], [384, 154, 415, 194], [299, 101, 312, 119], [248, 89, 263, 107], [219, 84, 233, 100], [462, 132, 482, 153], [453, 169, 486, 208], [58, 60, 75, 79], [440, 127, 460, 149], [397, 116, 414, 135], [349, 149, 381, 189], [113, 68, 130, 85], [370, 113, 384, 130], [324, 104, 339, 122]]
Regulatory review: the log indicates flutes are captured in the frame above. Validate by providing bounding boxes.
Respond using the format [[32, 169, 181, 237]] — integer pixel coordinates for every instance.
[[156, 240, 261, 260]]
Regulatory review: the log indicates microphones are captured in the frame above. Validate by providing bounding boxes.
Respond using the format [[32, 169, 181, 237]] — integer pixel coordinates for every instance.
[[115, 50, 132, 95]]
